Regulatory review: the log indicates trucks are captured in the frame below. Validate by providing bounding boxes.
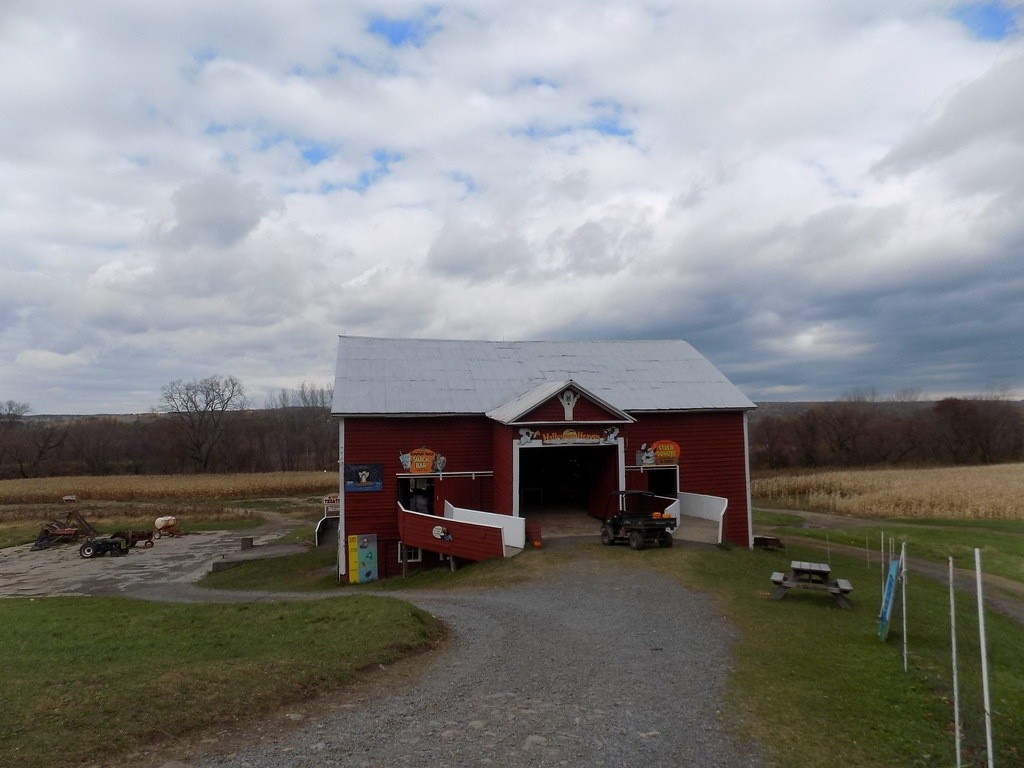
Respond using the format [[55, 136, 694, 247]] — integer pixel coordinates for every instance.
[[600, 491, 677, 550]]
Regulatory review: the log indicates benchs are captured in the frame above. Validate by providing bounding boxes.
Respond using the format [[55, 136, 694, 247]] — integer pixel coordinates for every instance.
[[769, 572, 854, 594]]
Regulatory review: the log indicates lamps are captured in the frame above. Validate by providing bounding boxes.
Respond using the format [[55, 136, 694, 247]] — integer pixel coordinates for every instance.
[[640, 443, 649, 453]]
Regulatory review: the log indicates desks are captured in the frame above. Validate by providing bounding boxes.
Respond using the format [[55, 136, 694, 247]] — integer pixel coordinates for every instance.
[[769, 561, 853, 603]]
